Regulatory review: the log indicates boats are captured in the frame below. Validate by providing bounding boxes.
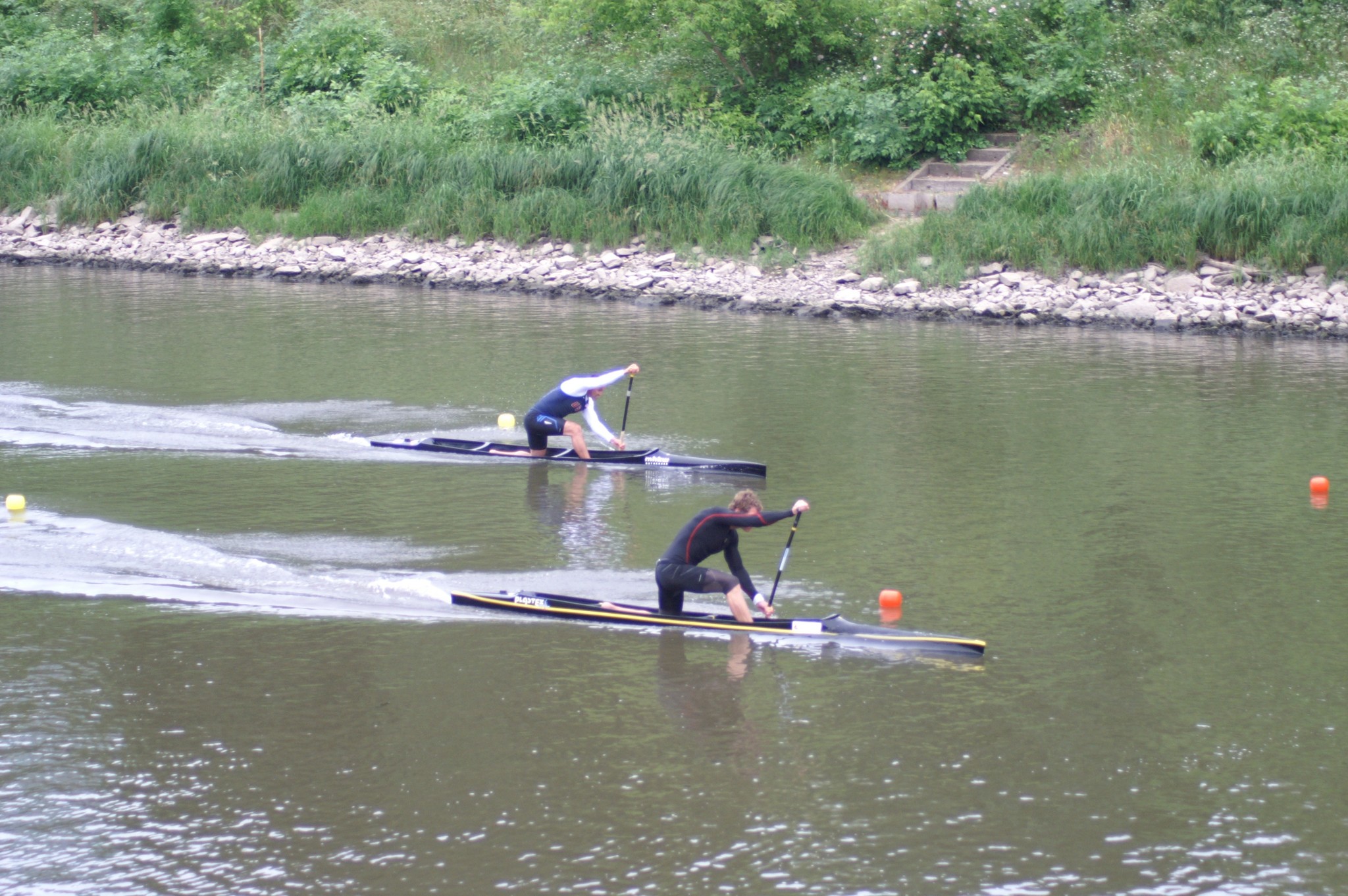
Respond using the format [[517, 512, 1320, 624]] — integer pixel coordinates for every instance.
[[451, 592, 987, 652], [370, 437, 767, 477]]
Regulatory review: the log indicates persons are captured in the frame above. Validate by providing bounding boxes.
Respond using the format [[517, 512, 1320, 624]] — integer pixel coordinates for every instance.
[[654, 624, 754, 766], [525, 461, 632, 550], [489, 363, 639, 458], [600, 487, 809, 622]]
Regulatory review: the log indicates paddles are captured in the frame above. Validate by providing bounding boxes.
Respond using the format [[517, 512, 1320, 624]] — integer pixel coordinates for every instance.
[[766, 507, 802, 619], [619, 369, 635, 450]]
